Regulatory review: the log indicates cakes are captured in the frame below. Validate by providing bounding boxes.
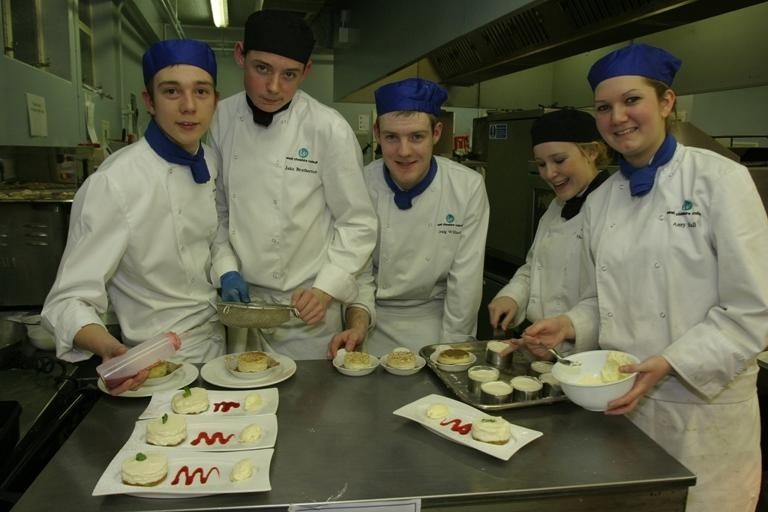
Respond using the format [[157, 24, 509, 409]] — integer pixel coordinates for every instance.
[[170, 387, 210, 412], [122, 451, 167, 488], [238, 350, 266, 372], [146, 356, 181, 380], [343, 352, 370, 369], [439, 348, 468, 363], [470, 416, 512, 445], [387, 351, 414, 367], [146, 414, 187, 445]]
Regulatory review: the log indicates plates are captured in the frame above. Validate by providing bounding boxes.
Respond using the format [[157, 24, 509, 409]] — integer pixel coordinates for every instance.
[[428, 344, 476, 372], [89, 387, 277, 502], [392, 393, 543, 462], [201, 353, 297, 389], [380, 345, 426, 375], [333, 349, 379, 377], [97, 363, 200, 399]]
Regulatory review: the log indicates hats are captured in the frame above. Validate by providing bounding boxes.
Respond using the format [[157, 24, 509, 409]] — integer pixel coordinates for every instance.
[[532, 108, 596, 146], [246, 9, 316, 64], [142, 40, 217, 90], [374, 79, 450, 116], [587, 45, 683, 90]]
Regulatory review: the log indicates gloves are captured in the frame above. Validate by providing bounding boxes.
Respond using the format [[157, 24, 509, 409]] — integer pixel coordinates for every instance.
[[219, 271, 251, 304]]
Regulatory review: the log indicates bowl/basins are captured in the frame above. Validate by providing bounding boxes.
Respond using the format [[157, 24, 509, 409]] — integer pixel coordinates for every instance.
[[552, 349, 643, 414]]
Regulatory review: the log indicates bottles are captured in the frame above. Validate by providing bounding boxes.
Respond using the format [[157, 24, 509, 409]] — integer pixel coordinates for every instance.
[[98, 331, 191, 388]]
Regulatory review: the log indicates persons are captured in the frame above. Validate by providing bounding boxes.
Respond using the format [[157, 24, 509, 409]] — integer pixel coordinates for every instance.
[[203, 9, 381, 361], [327, 78, 492, 360], [39, 39, 229, 397], [521, 44, 767, 512], [488, 108, 611, 358]]
